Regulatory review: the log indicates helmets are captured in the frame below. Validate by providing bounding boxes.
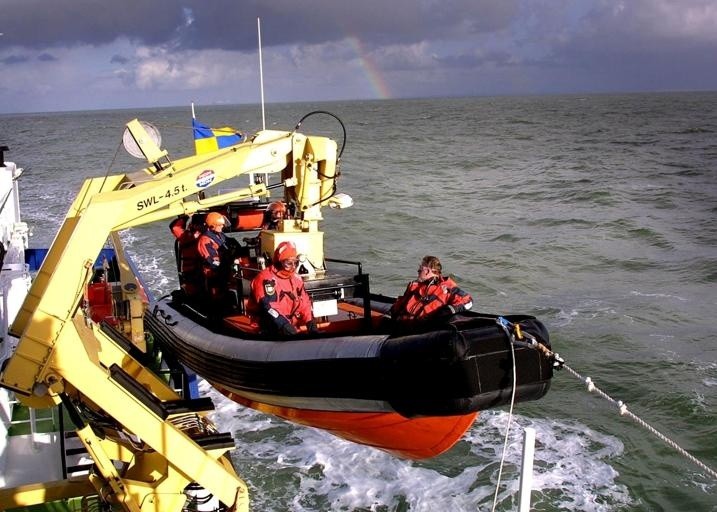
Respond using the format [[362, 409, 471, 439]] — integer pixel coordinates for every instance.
[[274, 242, 298, 263], [206, 212, 226, 230], [264, 202, 285, 218]]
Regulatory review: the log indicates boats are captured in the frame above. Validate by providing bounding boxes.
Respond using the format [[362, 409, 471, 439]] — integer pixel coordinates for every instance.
[[143, 290, 555, 463], [0, 144, 254, 512]]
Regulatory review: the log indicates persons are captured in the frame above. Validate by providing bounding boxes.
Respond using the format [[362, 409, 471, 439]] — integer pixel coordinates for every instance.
[[250, 242, 318, 340], [377, 256, 474, 334], [255, 201, 286, 241], [168, 211, 250, 332]]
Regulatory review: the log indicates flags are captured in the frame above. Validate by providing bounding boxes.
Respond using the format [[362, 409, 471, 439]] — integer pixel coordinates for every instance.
[[192, 117, 247, 153]]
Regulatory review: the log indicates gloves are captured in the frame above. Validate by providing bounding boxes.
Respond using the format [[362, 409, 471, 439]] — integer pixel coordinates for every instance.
[[306, 320, 318, 332], [436, 304, 455, 317]]
